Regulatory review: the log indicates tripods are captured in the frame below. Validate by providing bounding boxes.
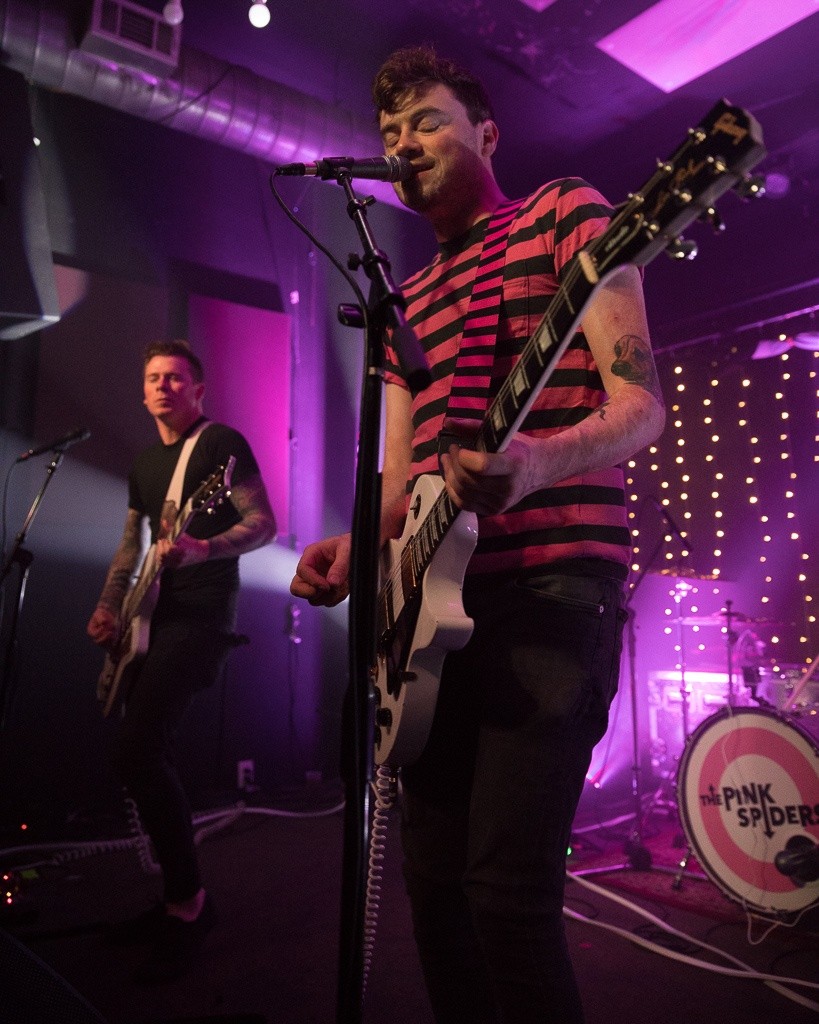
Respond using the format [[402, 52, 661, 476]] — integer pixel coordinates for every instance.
[[563, 527, 711, 880]]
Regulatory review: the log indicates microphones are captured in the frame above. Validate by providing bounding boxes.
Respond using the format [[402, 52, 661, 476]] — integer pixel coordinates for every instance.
[[16, 425, 91, 464], [277, 156, 413, 183], [654, 497, 694, 552], [774, 845, 819, 876]]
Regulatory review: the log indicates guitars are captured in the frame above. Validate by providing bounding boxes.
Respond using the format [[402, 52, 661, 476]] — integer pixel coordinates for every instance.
[[98, 452, 239, 723], [369, 89, 775, 771]]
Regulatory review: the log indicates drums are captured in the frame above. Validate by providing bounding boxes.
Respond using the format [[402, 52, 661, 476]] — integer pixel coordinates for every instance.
[[675, 704, 819, 918], [768, 669, 819, 712]]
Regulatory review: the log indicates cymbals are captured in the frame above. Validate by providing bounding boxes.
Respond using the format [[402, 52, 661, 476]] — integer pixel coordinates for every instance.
[[661, 609, 795, 627]]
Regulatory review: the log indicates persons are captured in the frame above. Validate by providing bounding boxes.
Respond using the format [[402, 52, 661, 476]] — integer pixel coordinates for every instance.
[[287, 44, 665, 1024], [86, 339, 278, 962]]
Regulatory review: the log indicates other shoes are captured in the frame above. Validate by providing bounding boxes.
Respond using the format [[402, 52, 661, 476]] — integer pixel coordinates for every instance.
[[148, 887, 217, 980], [104, 904, 163, 943]]
